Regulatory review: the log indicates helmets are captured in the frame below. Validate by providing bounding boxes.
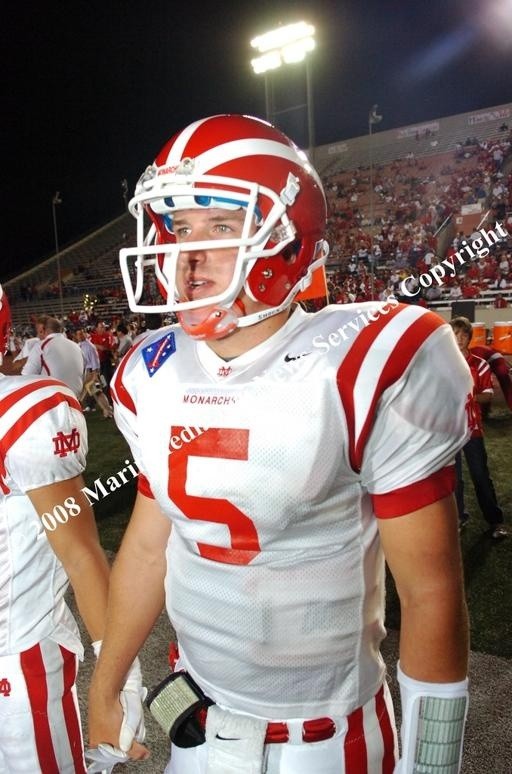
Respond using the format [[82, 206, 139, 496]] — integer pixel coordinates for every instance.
[[142, 114, 330, 308], [0, 281, 13, 355]]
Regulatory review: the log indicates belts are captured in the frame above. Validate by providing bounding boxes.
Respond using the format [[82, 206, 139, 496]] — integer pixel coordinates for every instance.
[[166, 641, 347, 745]]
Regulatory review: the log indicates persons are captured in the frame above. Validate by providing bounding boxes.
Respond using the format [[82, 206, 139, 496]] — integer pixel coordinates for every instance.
[[85, 112, 485, 772], [4, 117, 511, 544], [0, 281, 153, 771]]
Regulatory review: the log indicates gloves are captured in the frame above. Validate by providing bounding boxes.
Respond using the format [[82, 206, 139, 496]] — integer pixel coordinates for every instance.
[[81, 637, 153, 774]]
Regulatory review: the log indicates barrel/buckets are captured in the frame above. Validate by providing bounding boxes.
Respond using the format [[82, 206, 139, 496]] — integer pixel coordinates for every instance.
[[491, 321, 511, 356], [468, 320, 488, 350]]
